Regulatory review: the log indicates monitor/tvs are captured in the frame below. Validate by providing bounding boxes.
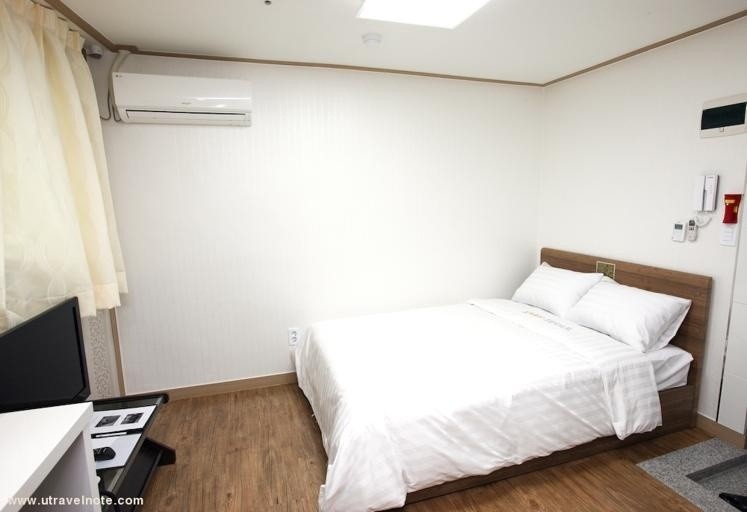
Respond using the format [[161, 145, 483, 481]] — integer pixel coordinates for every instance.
[[0, 294, 91, 412]]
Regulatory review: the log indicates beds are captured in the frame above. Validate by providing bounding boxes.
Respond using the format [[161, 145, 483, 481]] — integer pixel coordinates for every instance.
[[291, 246, 713, 511]]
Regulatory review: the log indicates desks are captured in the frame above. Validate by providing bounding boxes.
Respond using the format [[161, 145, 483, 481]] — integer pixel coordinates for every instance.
[[87, 393, 178, 510]]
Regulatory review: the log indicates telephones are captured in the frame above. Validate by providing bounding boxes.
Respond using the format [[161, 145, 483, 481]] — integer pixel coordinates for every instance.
[[694, 174, 719, 214]]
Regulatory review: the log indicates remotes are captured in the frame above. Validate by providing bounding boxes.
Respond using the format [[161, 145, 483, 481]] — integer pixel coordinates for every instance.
[[93, 446, 115, 461]]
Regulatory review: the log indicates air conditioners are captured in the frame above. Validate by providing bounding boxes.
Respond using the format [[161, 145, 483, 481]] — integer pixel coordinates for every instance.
[[106, 48, 253, 127]]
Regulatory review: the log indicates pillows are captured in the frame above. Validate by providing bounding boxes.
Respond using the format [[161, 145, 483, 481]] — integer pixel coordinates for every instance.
[[511, 262, 693, 355]]
